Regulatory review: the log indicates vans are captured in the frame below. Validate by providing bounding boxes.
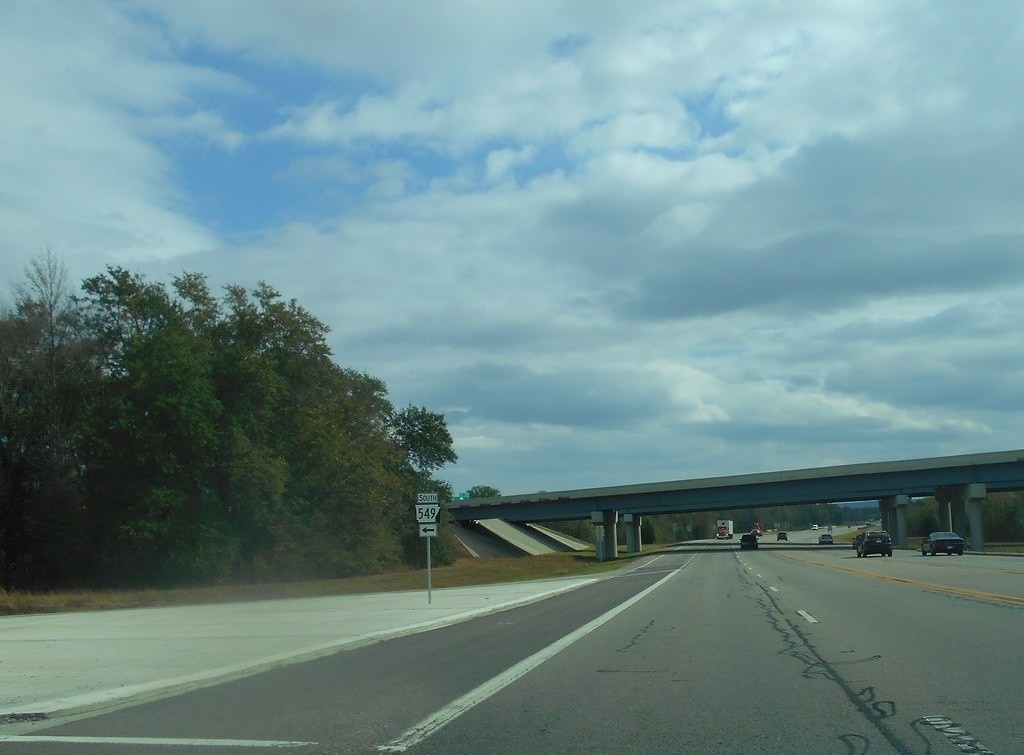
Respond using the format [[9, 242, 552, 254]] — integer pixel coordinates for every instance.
[[811, 524, 819, 530]]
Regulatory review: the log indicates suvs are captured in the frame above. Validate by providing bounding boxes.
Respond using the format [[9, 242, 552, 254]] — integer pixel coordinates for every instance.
[[856, 530, 892, 557]]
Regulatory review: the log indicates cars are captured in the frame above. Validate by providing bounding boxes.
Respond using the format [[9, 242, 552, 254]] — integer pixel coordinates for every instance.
[[921, 532, 964, 556], [777, 531, 788, 541], [818, 534, 833, 545], [852, 535, 862, 550], [739, 534, 759, 550]]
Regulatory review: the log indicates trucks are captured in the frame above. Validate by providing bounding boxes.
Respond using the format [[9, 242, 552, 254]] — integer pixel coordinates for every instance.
[[750, 522, 763, 536]]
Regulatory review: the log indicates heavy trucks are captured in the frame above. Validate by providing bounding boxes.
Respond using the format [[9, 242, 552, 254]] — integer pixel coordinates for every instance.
[[716, 519, 733, 539]]
